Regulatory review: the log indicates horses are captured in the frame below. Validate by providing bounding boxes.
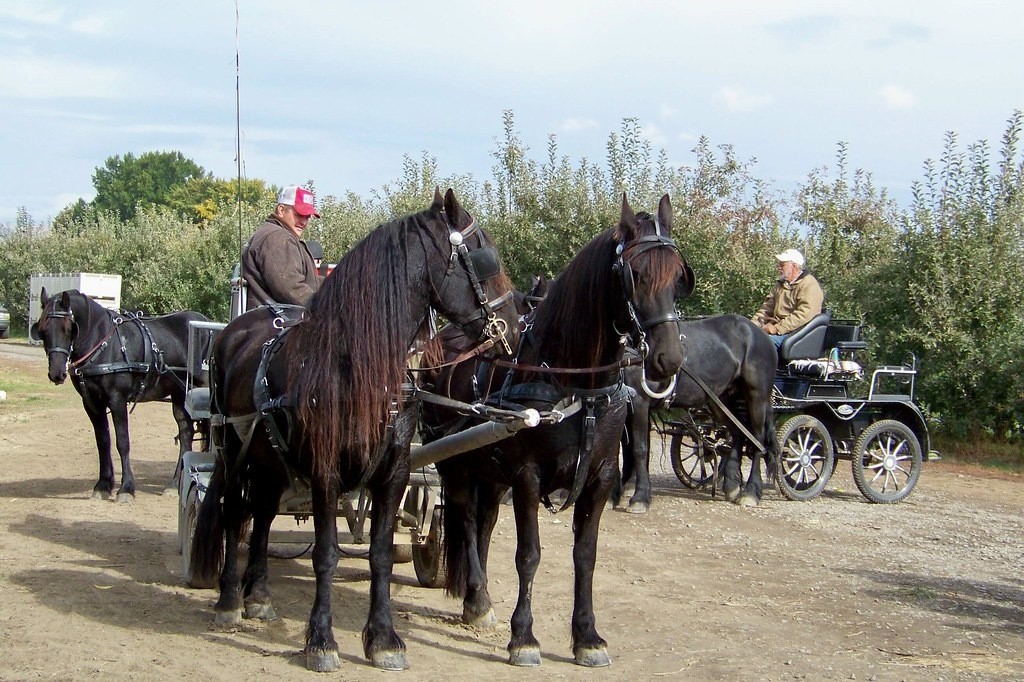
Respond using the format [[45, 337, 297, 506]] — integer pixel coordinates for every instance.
[[191, 187, 686, 674], [37, 285, 216, 504], [529, 271, 783, 512]]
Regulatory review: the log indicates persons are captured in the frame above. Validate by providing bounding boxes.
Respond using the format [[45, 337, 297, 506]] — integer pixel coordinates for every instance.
[[241, 187, 320, 312], [751, 250, 824, 405]]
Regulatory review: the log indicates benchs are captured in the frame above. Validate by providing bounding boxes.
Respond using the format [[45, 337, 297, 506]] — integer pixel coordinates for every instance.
[[230, 262, 339, 320]]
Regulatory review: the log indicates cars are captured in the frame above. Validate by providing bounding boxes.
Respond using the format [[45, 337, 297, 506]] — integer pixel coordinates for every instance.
[[0, 300, 11, 339]]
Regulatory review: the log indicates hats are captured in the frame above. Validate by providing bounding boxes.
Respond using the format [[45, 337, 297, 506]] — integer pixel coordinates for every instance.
[[278, 185, 320, 219], [774, 249, 805, 267]]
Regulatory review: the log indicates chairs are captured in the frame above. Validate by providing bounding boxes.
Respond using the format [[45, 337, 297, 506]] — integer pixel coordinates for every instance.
[[781, 306, 831, 360]]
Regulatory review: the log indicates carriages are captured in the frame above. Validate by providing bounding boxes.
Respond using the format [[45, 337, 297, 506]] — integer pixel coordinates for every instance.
[[168, 189, 696, 673], [603, 307, 931, 507]]
